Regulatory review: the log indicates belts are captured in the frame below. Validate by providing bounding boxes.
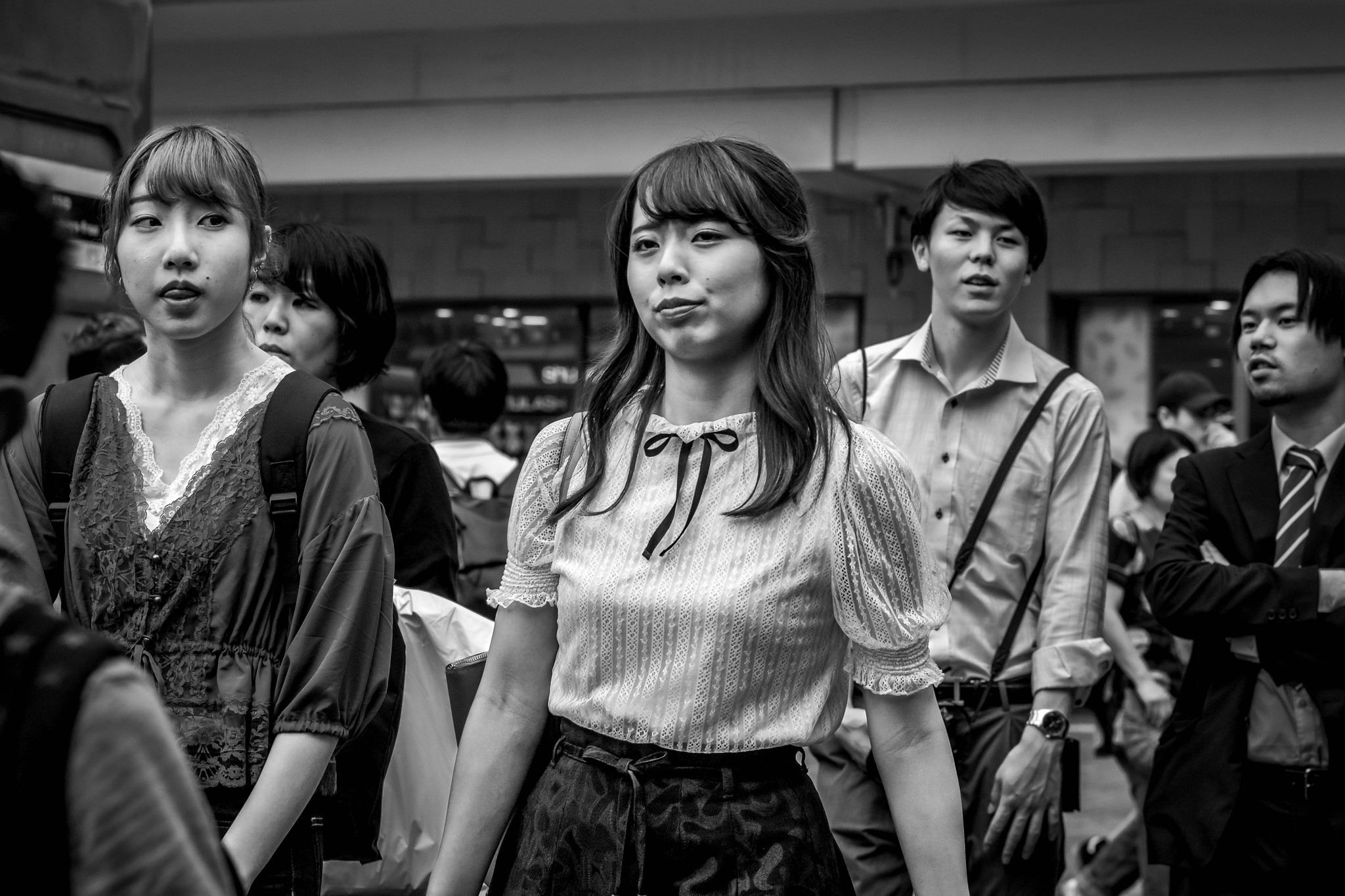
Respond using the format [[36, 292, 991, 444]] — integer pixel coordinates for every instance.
[[937, 684, 1032, 710], [1262, 766, 1340, 801]]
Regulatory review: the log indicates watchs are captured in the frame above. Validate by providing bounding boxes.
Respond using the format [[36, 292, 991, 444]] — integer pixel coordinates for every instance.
[[1025, 708, 1070, 742]]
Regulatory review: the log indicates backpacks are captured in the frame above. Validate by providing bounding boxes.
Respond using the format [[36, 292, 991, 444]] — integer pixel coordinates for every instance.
[[434, 462, 524, 620], [38, 368, 455, 868]]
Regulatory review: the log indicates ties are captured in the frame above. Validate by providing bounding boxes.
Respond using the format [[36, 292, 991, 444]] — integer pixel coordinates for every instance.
[[1274, 447, 1321, 569]]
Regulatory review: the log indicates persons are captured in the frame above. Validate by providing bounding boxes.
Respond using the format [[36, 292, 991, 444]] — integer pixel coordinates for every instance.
[[809, 154, 1112, 896], [1057, 374, 1239, 896], [426, 136, 971, 896], [242, 224, 460, 604], [1141, 248, 1345, 896], [429, 342, 521, 622], [0, 119, 395, 895], [0, 153, 249, 896]]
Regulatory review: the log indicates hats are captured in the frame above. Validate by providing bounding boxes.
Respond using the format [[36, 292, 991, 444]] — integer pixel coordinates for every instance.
[[1151, 369, 1234, 419]]
